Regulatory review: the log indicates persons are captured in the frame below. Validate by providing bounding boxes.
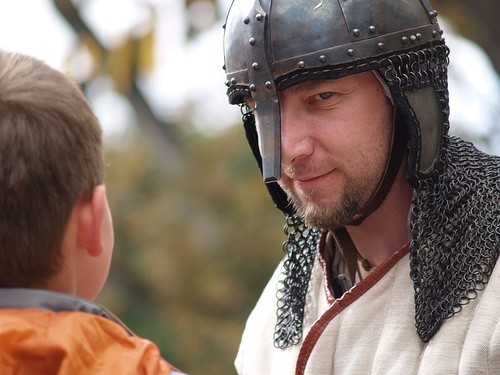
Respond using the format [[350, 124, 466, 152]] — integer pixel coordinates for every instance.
[[0, 49, 194, 375], [222, 0, 500, 375]]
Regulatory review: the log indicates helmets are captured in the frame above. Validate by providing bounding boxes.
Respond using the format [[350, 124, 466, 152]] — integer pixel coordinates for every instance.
[[221, 0, 450, 223]]
